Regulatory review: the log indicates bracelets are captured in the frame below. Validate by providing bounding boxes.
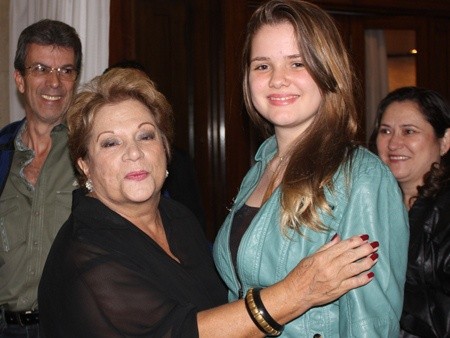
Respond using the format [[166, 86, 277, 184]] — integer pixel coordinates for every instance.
[[244, 288, 284, 337]]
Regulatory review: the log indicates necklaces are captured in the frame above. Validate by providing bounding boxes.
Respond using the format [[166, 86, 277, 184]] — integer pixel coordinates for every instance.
[[275, 153, 292, 166]]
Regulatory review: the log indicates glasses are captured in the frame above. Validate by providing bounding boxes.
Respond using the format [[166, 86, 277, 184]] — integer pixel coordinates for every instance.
[[24, 63, 80, 81]]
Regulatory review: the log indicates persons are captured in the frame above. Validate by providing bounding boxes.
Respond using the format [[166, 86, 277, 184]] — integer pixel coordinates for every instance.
[[369, 86, 450, 338], [37, 67, 379, 338], [0, 18, 82, 338], [212, 0, 410, 338]]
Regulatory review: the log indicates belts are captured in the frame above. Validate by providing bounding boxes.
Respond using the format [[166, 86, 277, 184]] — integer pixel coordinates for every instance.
[[0, 309, 40, 325]]
